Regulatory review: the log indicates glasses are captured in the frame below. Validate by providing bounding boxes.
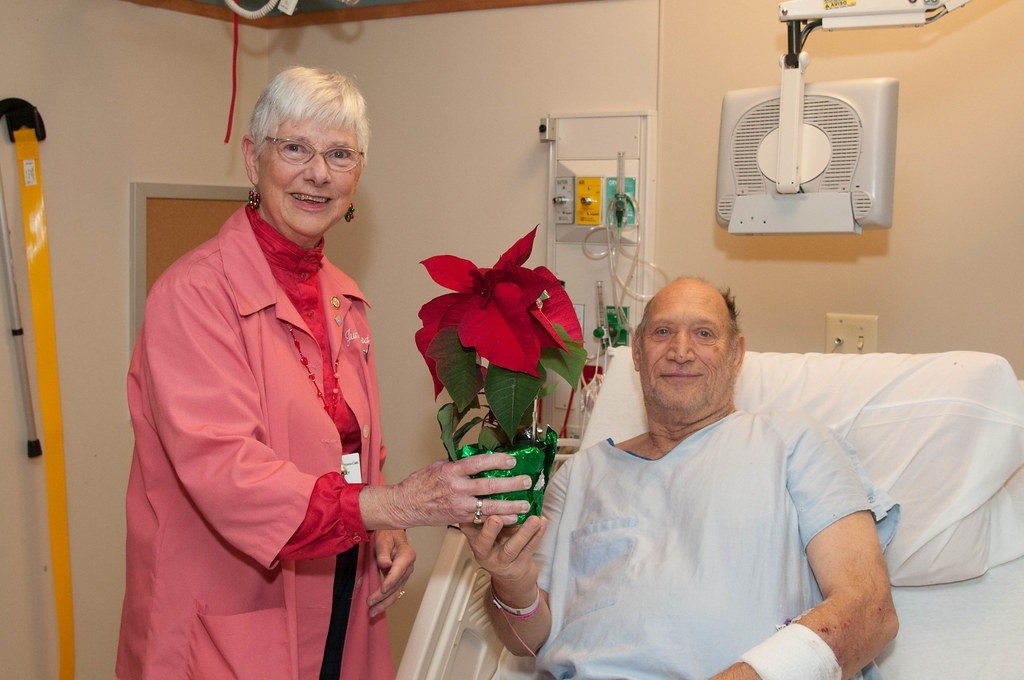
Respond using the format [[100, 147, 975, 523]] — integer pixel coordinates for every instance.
[[266, 135, 363, 173]]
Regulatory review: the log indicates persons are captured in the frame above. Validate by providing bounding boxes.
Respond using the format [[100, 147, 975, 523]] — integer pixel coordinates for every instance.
[[113, 72, 531, 679], [286, 322, 348, 477], [460, 277, 901, 680]]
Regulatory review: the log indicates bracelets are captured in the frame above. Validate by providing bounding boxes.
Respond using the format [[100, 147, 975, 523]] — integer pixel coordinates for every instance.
[[490, 577, 541, 659], [399, 591, 406, 599]]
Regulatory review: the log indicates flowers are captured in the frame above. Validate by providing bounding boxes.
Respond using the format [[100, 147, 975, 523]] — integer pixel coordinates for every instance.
[[415, 223, 587, 466]]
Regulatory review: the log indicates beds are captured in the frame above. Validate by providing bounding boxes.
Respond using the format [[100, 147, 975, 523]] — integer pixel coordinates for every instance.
[[395, 345, 1024, 680]]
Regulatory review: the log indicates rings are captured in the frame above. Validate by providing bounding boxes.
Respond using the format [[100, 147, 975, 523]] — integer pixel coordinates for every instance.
[[474, 516, 481, 523], [475, 498, 483, 516]]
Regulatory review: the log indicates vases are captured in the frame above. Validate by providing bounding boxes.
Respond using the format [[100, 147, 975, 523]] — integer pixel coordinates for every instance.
[[455, 426, 558, 528]]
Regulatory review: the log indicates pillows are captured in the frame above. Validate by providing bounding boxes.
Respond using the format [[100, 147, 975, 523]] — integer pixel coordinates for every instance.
[[580, 345, 1024, 584]]
